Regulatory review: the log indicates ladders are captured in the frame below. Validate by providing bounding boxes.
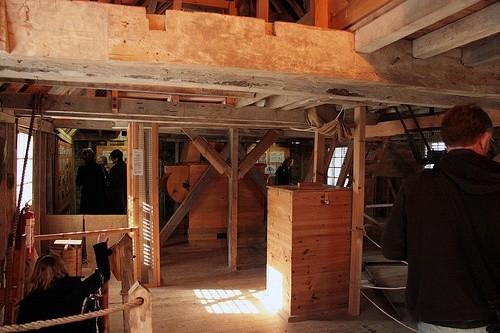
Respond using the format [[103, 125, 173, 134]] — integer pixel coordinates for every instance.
[[394, 105, 433, 170]]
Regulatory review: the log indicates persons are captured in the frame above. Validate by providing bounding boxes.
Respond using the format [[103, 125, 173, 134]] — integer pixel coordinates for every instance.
[[16, 241, 110, 333], [274, 157, 295, 186], [381, 101, 500, 333], [75, 147, 126, 214]]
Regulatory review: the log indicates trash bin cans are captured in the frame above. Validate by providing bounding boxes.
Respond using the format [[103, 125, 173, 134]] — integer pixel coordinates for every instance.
[[50, 240, 81, 282]]
